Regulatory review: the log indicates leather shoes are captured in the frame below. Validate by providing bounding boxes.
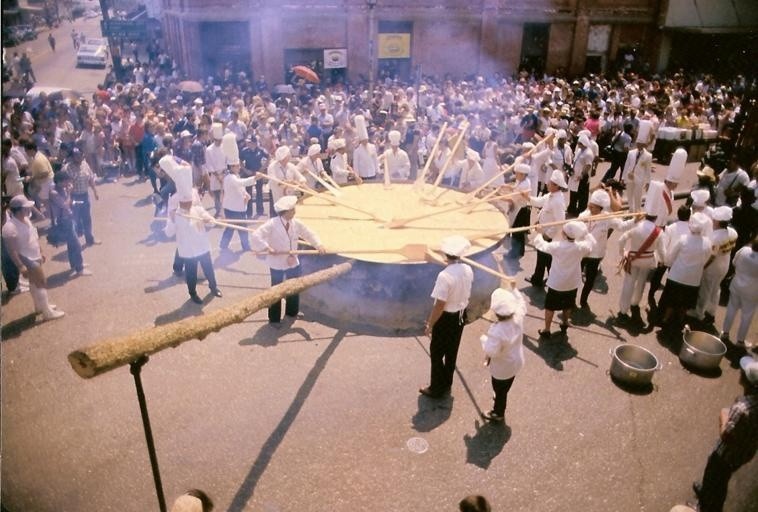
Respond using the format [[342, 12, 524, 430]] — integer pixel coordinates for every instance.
[[537, 329, 550, 336]]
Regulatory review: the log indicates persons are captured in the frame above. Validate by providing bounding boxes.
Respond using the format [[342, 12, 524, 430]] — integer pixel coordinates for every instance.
[[480, 278, 526, 423], [686, 355, 758, 512], [458, 493, 492, 511], [171, 488, 213, 512], [0, 22, 758, 346], [419, 237, 474, 398]]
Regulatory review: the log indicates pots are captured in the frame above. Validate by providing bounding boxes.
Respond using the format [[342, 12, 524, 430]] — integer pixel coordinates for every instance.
[[294, 180, 510, 268], [608, 344, 663, 388], [678, 329, 727, 370]]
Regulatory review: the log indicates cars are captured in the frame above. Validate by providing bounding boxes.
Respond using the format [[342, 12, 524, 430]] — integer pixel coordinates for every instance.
[[1, 85, 84, 107], [3, 25, 37, 47], [75, 38, 110, 67]]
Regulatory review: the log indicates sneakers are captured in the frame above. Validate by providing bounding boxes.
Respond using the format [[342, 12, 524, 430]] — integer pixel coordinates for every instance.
[[8, 280, 30, 295], [212, 286, 221, 297], [175, 269, 183, 276], [525, 277, 532, 281], [296, 311, 304, 318], [686, 501, 699, 511], [92, 239, 102, 244], [269, 320, 282, 329], [192, 295, 202, 304], [72, 263, 92, 275], [480, 410, 505, 421], [419, 386, 451, 396], [617, 299, 715, 333], [34, 303, 65, 321], [719, 329, 752, 348], [693, 480, 703, 494]]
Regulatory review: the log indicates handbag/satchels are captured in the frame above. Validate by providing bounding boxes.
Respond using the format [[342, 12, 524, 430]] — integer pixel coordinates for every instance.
[[46, 220, 74, 244]]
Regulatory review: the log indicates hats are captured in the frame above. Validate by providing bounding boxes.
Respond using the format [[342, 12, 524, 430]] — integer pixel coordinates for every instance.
[[696, 166, 716, 181], [466, 147, 480, 161], [245, 135, 257, 142], [308, 143, 321, 156], [666, 148, 688, 184], [555, 129, 567, 140], [523, 141, 537, 152], [743, 187, 758, 200], [490, 287, 518, 317], [354, 115, 369, 141], [644, 180, 665, 217], [419, 86, 427, 93], [590, 189, 611, 208], [514, 164, 532, 175], [142, 88, 151, 95], [713, 206, 733, 221], [317, 94, 342, 110], [331, 138, 346, 150], [212, 122, 223, 140], [689, 211, 710, 233], [273, 196, 298, 213], [389, 130, 401, 146], [222, 133, 240, 165], [180, 129, 193, 142], [159, 155, 193, 203], [551, 169, 569, 189], [10, 194, 35, 208], [275, 145, 290, 161], [739, 354, 758, 384], [636, 119, 652, 144], [577, 130, 591, 147], [441, 236, 471, 256], [562, 221, 587, 240], [72, 146, 82, 155], [170, 95, 204, 105], [690, 188, 710, 203]]
